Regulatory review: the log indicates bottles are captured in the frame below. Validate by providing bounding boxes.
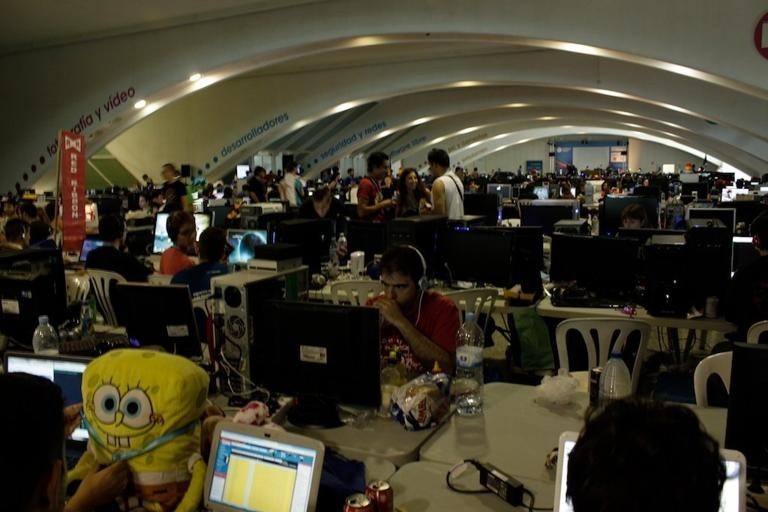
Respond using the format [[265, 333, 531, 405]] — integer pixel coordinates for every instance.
[[451, 313, 484, 415], [350, 250, 364, 272], [330, 232, 348, 265], [598, 351, 633, 415], [32, 314, 59, 351], [591, 215, 599, 234]]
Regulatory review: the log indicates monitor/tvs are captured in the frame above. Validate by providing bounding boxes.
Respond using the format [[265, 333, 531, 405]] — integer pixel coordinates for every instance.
[[3, 350, 96, 451], [150, 211, 216, 257], [1, 247, 68, 350], [518, 197, 581, 237], [251, 298, 383, 431], [460, 170, 768, 312], [112, 281, 204, 363], [181, 165, 252, 212], [225, 228, 270, 267]]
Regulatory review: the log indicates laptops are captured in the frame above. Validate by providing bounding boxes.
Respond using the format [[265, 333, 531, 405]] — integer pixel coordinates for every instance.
[[717, 447, 747, 511], [201, 421, 325, 512], [552, 430, 576, 512], [69, 239, 115, 270]]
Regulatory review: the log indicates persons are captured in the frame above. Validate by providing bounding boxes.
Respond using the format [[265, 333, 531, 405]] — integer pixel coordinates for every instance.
[[1, 149, 767, 511]]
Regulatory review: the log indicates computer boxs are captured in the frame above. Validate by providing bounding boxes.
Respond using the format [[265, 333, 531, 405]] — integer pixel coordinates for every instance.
[[391, 214, 488, 284], [512, 183, 521, 199], [670, 180, 683, 198], [463, 193, 503, 226], [665, 203, 685, 230], [697, 175, 712, 200], [585, 180, 605, 206], [240, 201, 332, 289], [211, 262, 310, 402], [87, 192, 128, 238], [552, 219, 589, 238], [542, 180, 552, 197], [126, 224, 154, 257]]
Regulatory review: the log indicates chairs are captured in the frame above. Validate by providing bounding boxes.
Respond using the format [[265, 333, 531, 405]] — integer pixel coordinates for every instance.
[[65, 271, 90, 305], [87, 269, 127, 326]]
